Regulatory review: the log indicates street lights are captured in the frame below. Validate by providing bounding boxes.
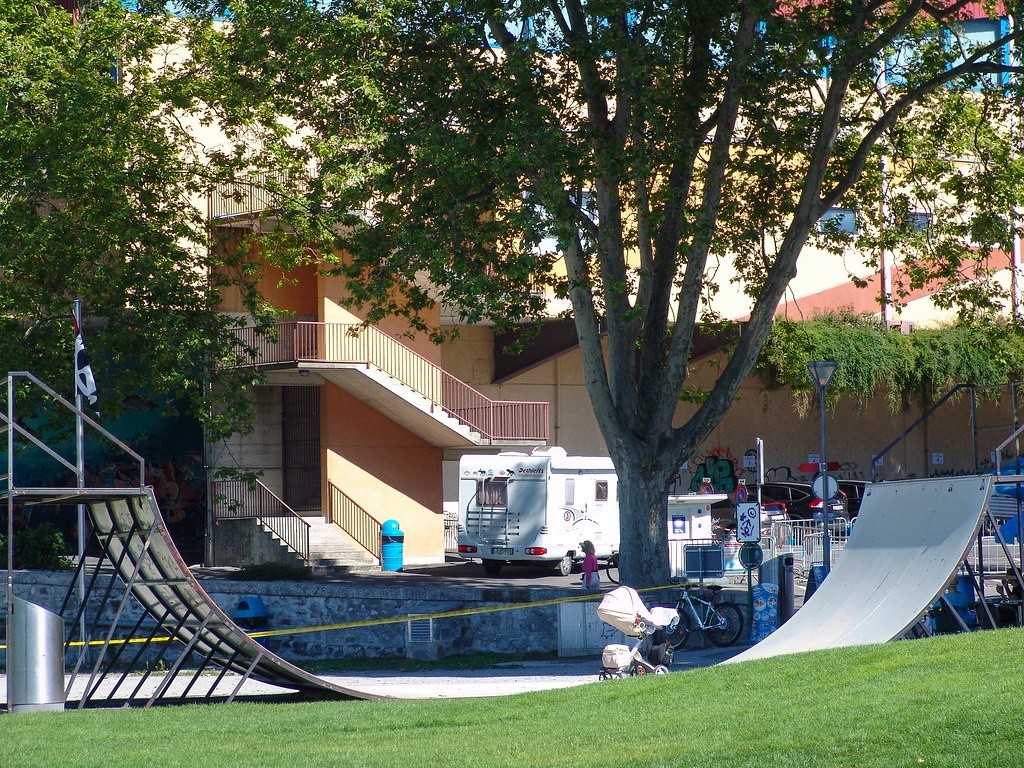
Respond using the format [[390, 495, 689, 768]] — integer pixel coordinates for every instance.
[[806, 360, 839, 575]]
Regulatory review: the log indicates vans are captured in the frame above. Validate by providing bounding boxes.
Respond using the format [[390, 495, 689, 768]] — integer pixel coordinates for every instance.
[[456, 445, 619, 577]]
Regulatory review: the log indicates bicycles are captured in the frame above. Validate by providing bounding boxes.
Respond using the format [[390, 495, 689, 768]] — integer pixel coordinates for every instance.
[[654, 584, 744, 651], [606, 549, 620, 584]]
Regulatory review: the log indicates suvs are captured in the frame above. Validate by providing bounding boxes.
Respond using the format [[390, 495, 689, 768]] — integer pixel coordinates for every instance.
[[711, 478, 872, 546]]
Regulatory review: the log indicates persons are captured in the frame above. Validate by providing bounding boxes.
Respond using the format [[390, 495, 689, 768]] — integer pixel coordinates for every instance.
[[996, 568, 1024, 602], [580, 541, 601, 589]]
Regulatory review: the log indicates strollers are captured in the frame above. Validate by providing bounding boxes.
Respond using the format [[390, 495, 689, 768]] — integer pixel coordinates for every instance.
[[596, 584, 681, 681]]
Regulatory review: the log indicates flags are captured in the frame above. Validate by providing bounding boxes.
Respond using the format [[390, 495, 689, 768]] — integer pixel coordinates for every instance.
[[71, 309, 100, 418]]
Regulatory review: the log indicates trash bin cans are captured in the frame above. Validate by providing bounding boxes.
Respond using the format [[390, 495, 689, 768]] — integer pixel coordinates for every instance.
[[379, 519, 404, 572], [930, 575, 977, 637], [758, 553, 794, 629], [7, 595, 67, 712]]
